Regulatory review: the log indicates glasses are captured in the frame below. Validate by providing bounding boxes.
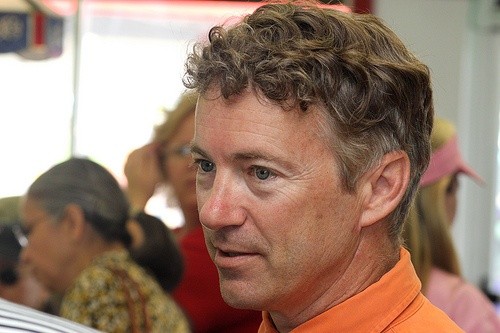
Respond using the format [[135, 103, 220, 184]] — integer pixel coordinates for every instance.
[[11, 209, 64, 246]]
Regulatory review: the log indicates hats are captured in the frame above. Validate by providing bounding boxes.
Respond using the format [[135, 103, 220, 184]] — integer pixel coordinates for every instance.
[[421, 135, 486, 188]]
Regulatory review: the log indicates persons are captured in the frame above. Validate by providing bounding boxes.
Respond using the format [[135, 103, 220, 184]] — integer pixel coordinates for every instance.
[[182, 0, 463, 333], [125, 94, 263, 333], [16, 157, 190, 333], [398, 121, 500, 333]]
